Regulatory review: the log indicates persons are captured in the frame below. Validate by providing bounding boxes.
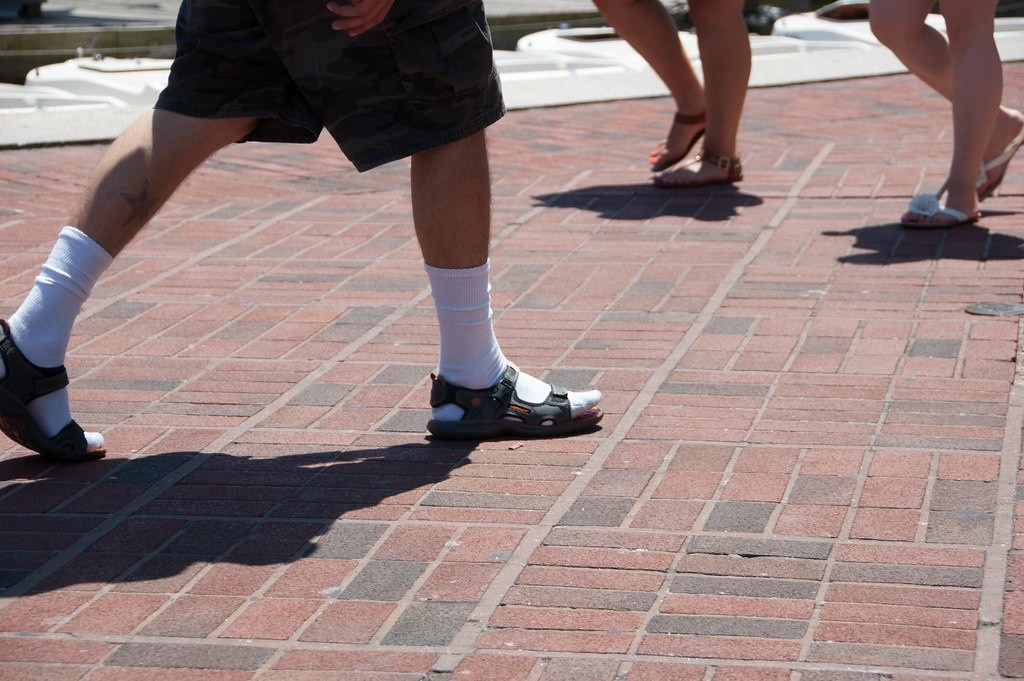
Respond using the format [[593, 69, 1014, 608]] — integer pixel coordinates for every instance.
[[0, 1, 607, 459], [592, 0, 751, 187], [868, 1, 1024, 228]]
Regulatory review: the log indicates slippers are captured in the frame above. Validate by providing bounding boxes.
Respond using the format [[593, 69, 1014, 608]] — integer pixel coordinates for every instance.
[[898, 194, 983, 227], [975, 125, 1024, 203]]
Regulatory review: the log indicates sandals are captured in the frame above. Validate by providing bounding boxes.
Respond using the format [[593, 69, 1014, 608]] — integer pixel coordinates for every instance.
[[425, 359, 605, 437], [657, 111, 710, 164], [0, 315, 108, 464], [651, 148, 744, 188]]
